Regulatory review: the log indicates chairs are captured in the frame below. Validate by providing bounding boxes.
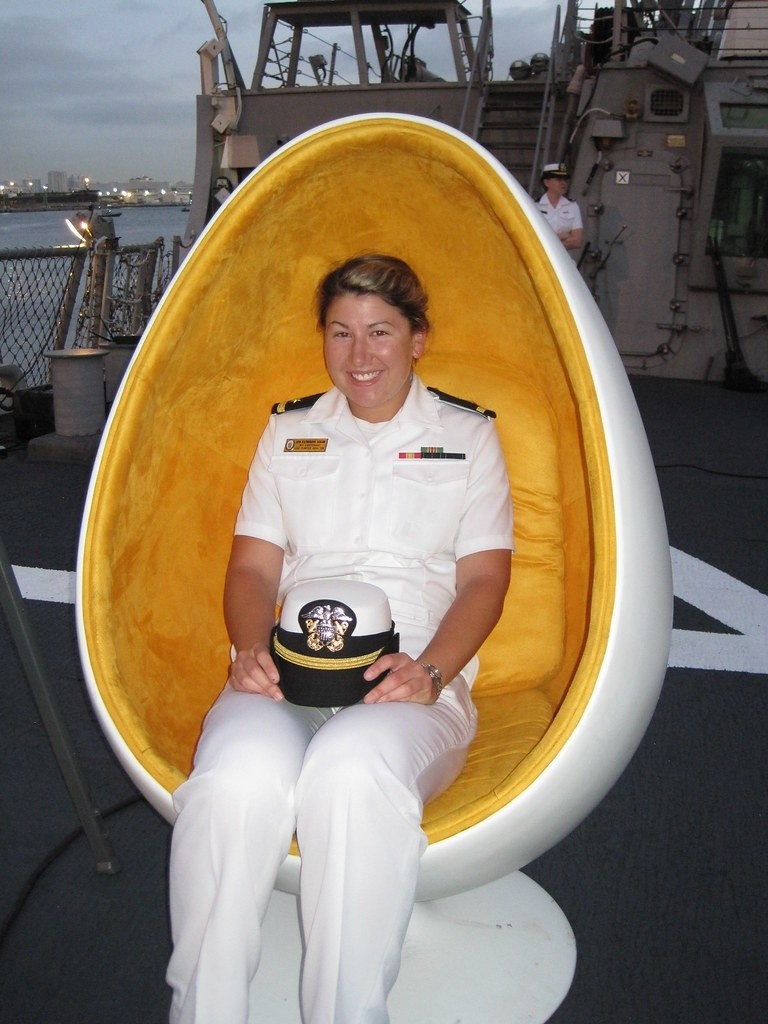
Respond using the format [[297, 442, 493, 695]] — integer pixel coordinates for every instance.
[[76, 112, 674, 1023]]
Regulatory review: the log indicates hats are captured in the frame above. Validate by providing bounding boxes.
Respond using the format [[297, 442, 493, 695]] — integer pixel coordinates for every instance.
[[539, 163, 572, 180], [272, 580, 402, 707]]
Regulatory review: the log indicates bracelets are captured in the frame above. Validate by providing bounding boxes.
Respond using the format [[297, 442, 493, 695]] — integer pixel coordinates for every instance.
[[415, 656, 444, 698]]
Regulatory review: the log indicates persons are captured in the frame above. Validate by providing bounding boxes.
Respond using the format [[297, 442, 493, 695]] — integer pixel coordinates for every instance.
[[165, 255, 513, 1024], [535, 163, 585, 257]]
